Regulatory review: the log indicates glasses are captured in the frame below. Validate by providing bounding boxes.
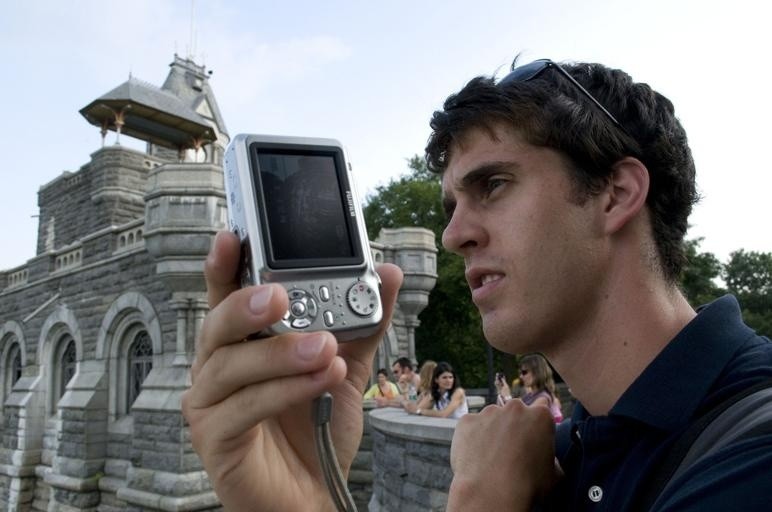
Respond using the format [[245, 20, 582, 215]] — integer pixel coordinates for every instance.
[[495, 58, 636, 141], [521, 368, 532, 375]]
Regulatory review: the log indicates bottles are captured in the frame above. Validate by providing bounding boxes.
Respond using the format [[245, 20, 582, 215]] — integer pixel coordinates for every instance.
[[408, 386, 418, 408]]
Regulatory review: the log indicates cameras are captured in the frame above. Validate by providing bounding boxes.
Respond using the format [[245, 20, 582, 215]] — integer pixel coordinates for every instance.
[[223, 133, 385, 341]]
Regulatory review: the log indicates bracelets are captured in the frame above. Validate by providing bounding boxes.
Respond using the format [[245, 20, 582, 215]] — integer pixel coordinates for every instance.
[[501, 396, 513, 399]]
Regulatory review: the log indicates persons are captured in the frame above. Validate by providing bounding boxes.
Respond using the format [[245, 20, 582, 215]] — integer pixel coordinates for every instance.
[[181, 56, 768, 511], [494, 354, 565, 424], [363, 357, 469, 421]]
